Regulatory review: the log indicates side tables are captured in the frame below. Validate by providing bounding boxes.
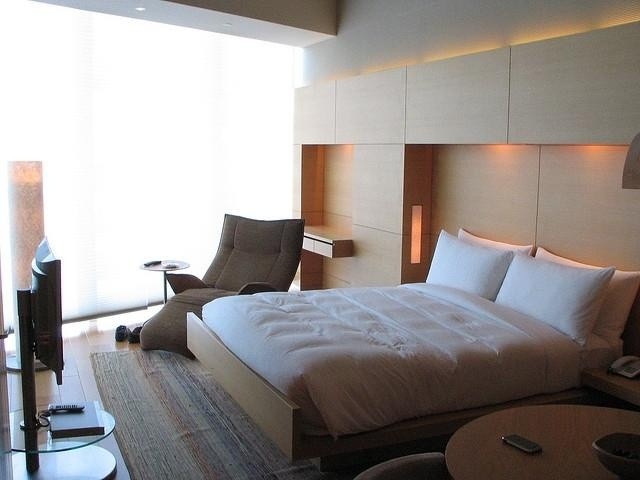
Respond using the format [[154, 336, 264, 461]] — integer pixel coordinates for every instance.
[[140, 260, 191, 306]]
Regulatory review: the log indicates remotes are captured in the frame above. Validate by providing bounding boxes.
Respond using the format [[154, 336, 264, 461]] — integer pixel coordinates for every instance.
[[144, 261, 161, 266], [48, 404, 85, 412]]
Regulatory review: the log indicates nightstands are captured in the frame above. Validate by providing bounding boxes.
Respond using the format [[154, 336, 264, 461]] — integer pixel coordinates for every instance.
[[581, 366, 640, 410]]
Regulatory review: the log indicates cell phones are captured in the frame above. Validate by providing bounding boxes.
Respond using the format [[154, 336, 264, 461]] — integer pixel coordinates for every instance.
[[503, 434, 542, 454]]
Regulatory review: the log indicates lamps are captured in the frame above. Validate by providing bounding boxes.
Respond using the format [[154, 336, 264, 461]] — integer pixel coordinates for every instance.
[[6, 160, 48, 373]]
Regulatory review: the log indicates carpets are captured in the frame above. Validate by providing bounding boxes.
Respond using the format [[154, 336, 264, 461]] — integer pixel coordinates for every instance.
[[90, 350, 329, 479]]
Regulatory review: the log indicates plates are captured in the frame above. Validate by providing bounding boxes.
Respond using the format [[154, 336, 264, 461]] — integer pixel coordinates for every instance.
[[161, 262, 180, 268]]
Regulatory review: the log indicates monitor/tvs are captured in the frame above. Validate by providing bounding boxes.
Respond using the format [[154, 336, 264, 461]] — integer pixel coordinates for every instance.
[[30, 238, 63, 386]]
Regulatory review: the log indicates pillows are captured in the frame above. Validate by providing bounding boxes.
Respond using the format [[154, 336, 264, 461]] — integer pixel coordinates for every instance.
[[426, 228, 640, 346]]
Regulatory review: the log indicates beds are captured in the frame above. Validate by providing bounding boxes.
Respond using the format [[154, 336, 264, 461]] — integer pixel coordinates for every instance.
[[185, 230, 640, 472]]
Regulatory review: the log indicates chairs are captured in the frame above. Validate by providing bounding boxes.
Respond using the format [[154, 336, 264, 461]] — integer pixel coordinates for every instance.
[[354, 452, 447, 479], [139, 214, 305, 359]]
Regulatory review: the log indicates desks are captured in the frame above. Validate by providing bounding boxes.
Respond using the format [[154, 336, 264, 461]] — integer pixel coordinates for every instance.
[[9, 406, 116, 480]]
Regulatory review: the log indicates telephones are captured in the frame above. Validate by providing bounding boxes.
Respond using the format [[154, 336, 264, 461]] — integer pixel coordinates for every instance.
[[609, 355, 640, 379]]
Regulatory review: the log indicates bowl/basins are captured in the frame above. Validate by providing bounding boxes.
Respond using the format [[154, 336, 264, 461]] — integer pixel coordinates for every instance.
[[593, 432, 639, 479]]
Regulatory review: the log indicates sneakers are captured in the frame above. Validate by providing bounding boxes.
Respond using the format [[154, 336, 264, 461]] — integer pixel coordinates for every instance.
[[116, 326, 141, 343]]
